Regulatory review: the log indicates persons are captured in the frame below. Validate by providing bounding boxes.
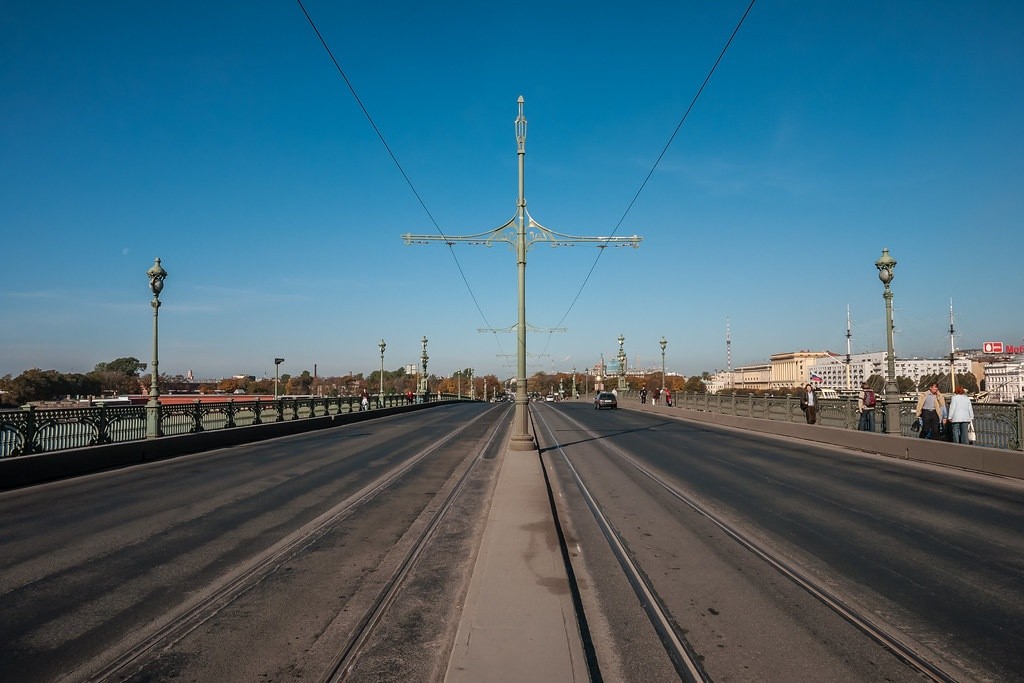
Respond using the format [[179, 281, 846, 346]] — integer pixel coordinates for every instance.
[[916, 382, 954, 442], [857, 382, 875, 432], [597, 389, 600, 396], [406, 390, 413, 404], [639, 386, 647, 404], [801, 384, 819, 425], [947, 386, 974, 445], [425, 390, 431, 402], [660, 387, 672, 406], [653, 387, 660, 403], [612, 387, 617, 398], [361, 388, 370, 411]]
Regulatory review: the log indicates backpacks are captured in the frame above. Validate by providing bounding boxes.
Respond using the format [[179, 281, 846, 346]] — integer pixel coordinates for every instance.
[[862, 391, 876, 407]]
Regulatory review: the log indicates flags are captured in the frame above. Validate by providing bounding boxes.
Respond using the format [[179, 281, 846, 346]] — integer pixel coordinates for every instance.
[[810, 372, 823, 382]]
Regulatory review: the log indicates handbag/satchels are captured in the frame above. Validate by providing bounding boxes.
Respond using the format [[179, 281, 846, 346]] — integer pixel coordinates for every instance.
[[801, 393, 808, 411], [911, 418, 920, 432], [967, 420, 976, 441]]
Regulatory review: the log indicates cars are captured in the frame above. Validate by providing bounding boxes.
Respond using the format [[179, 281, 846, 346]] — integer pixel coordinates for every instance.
[[593, 392, 617, 410], [545, 394, 554, 403], [495, 395, 503, 402]]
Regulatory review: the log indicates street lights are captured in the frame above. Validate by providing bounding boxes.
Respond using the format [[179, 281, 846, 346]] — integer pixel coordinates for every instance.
[[457, 370, 461, 399], [874, 248, 900, 435], [659, 336, 667, 402], [617, 334, 626, 388], [585, 368, 589, 395], [146, 255, 168, 439], [572, 367, 577, 396], [377, 339, 387, 407], [420, 336, 429, 401], [470, 368, 474, 400]]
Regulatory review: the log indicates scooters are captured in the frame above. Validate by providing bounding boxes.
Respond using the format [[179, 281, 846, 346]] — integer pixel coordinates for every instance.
[[360, 394, 370, 410]]
